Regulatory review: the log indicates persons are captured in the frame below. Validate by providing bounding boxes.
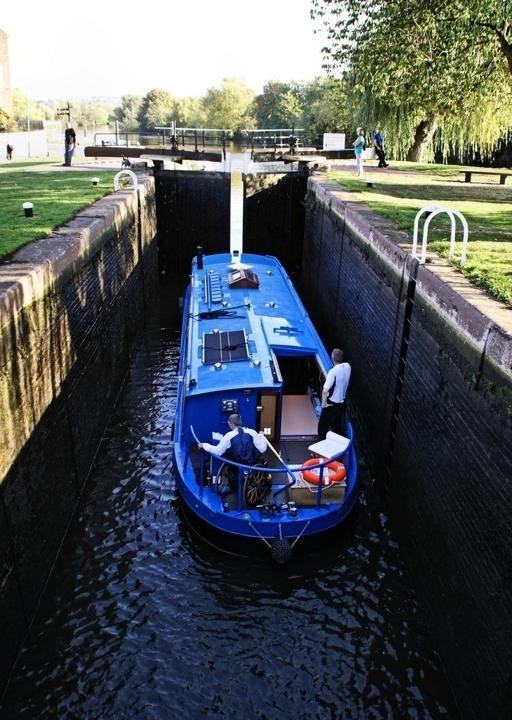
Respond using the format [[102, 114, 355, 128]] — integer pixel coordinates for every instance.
[[198, 414, 267, 491], [317, 348, 351, 440], [6, 141, 14, 160], [62, 122, 77, 166], [373, 127, 389, 168], [351, 127, 365, 177]]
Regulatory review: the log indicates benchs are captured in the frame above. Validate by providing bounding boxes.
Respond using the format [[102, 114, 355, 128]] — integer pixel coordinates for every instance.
[[459, 169, 512, 184]]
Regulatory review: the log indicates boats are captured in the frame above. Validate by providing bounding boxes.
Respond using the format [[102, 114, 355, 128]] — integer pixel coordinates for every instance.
[[173, 246, 358, 565]]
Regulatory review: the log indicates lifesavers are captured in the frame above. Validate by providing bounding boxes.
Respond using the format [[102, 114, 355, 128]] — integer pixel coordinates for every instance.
[[302, 458, 346, 485]]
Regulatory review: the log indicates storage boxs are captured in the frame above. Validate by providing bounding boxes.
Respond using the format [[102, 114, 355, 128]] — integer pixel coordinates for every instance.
[[287, 464, 347, 506]]
